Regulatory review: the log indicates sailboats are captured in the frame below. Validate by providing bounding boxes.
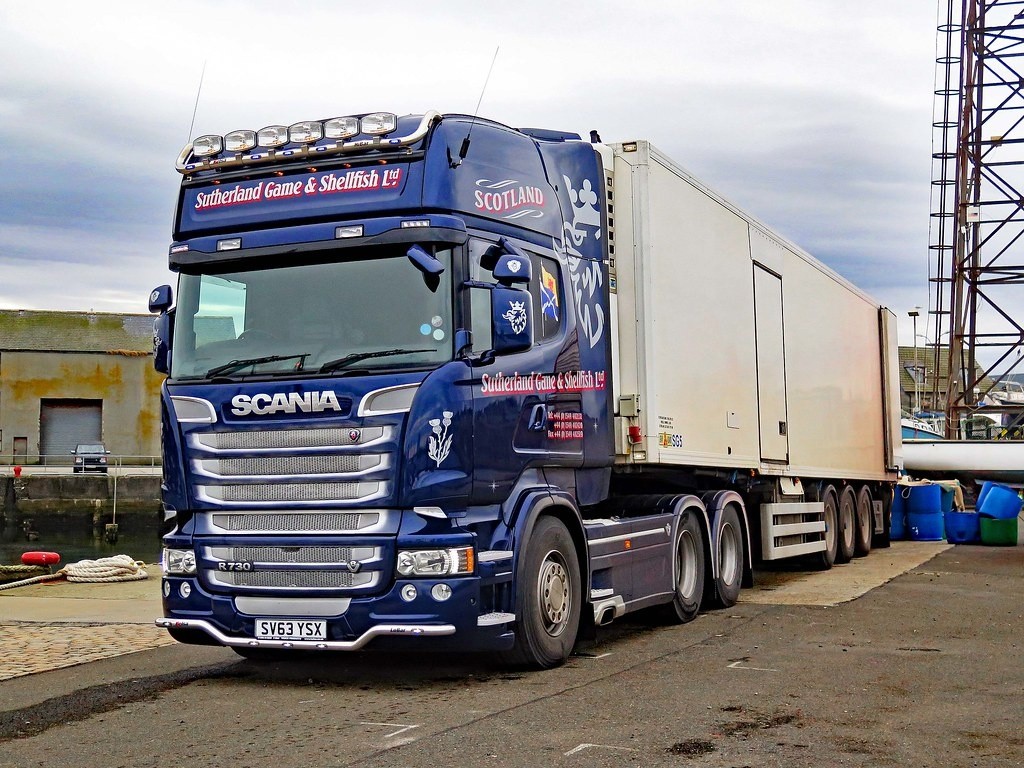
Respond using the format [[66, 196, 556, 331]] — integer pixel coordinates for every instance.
[[901, 1, 1024, 486]]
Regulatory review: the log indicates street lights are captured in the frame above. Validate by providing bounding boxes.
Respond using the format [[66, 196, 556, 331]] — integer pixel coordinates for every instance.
[[908, 310, 922, 413]]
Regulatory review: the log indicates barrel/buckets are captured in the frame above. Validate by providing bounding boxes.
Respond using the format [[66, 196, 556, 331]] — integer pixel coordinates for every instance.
[[891, 482, 1023, 547]]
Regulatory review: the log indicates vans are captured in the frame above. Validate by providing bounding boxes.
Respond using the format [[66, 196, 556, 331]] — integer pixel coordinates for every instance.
[[70, 441, 111, 473]]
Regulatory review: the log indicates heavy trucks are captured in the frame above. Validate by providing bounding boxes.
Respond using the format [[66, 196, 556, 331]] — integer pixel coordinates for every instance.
[[149, 111, 904, 675]]
